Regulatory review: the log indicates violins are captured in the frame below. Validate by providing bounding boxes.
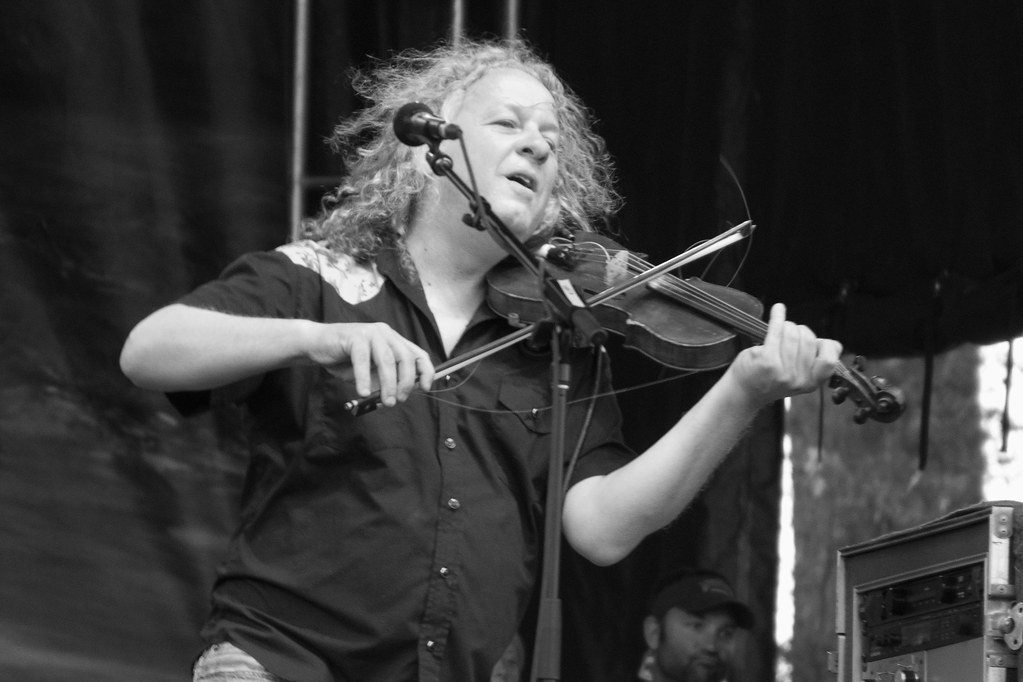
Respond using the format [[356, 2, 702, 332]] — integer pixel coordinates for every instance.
[[484, 227, 908, 425]]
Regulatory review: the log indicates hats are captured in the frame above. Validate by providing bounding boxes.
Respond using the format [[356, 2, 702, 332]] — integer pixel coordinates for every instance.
[[655, 575, 756, 630]]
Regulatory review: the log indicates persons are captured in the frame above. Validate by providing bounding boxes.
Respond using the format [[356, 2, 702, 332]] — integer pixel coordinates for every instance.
[[636, 569, 758, 682], [118, 41, 841, 682]]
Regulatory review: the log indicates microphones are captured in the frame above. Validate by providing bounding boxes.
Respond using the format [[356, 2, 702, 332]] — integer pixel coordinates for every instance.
[[393, 100, 463, 147]]
